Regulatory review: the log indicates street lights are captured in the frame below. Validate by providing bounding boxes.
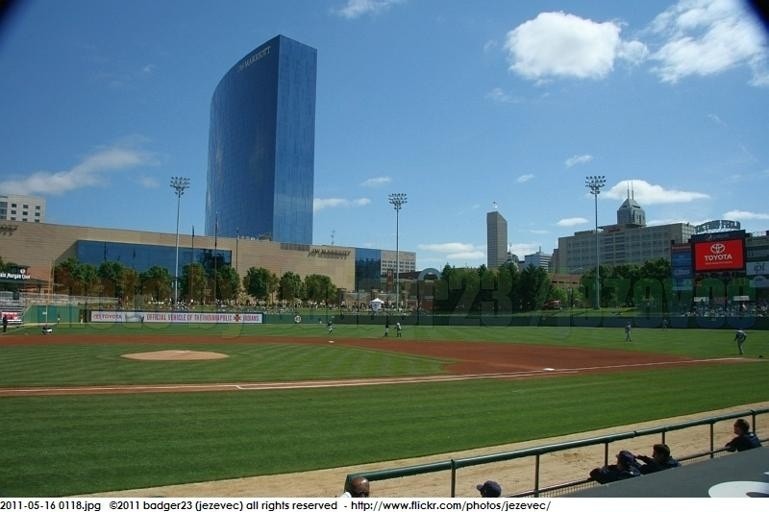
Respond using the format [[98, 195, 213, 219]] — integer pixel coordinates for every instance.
[[388, 193, 408, 309], [170, 176, 191, 303], [585, 175, 606, 310]]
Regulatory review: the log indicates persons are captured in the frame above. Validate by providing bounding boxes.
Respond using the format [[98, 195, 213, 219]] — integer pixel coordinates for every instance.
[[585, 450, 641, 485], [41, 324, 52, 336], [624, 320, 633, 342], [57, 313, 61, 323], [327, 319, 335, 336], [725, 419, 762, 452], [216, 299, 301, 316], [633, 444, 682, 475], [394, 322, 402, 337], [79, 312, 82, 323], [141, 317, 144, 328], [335, 477, 370, 497], [733, 327, 747, 354], [169, 296, 190, 312], [318, 317, 323, 324], [2, 314, 8, 332], [680, 297, 769, 318]]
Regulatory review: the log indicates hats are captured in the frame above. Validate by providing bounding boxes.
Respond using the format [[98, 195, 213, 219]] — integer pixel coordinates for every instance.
[[616, 450, 634, 466]]
